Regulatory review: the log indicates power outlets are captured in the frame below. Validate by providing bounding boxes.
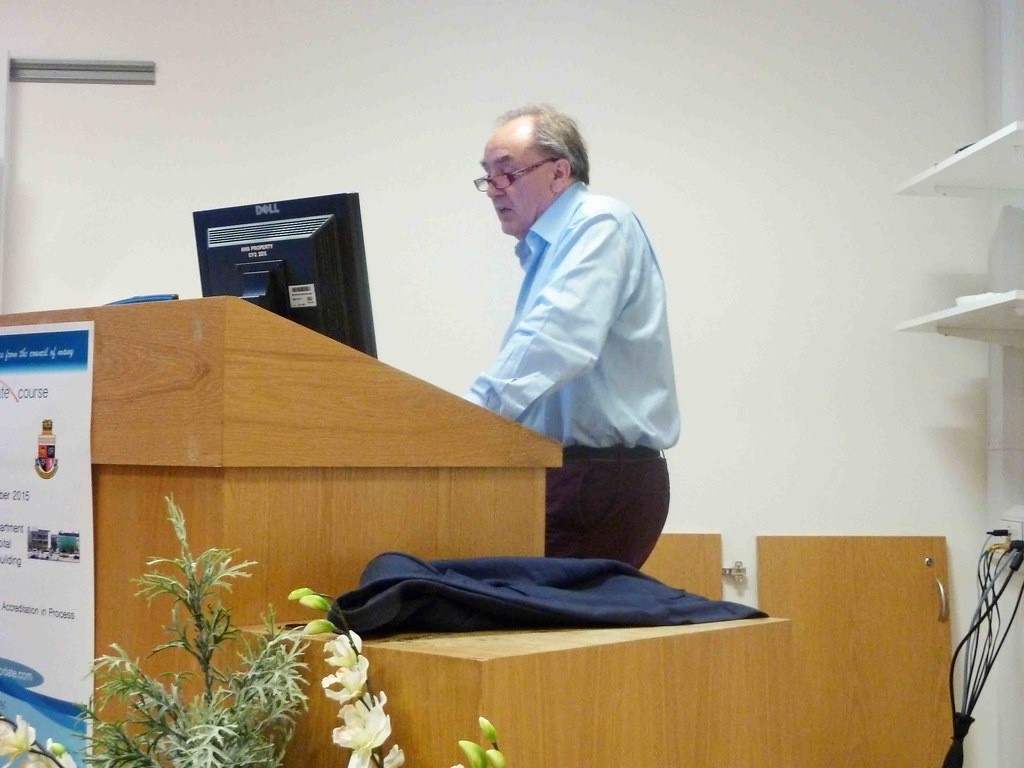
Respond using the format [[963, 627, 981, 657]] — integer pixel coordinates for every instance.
[[994, 519, 1024, 555]]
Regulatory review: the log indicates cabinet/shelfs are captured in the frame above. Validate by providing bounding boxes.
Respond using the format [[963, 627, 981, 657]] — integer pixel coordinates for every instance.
[[892, 119, 1024, 350]]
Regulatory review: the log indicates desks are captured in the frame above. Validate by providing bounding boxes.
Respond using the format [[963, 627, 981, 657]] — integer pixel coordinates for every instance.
[[228, 615, 805, 768]]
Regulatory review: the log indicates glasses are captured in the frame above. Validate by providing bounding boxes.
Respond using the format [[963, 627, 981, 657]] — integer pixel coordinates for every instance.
[[474, 158, 557, 191]]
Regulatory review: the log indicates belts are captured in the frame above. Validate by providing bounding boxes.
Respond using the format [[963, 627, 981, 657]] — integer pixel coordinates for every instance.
[[563, 447, 661, 464]]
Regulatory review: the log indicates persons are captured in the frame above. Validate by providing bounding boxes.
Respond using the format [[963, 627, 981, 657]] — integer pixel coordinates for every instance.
[[464, 104, 682, 570]]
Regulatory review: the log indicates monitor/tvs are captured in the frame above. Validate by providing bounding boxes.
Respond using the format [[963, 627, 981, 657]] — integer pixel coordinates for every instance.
[[192, 193, 379, 359]]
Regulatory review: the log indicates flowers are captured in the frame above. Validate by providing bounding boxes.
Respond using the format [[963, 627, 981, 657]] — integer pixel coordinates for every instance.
[[0, 490, 507, 768]]
